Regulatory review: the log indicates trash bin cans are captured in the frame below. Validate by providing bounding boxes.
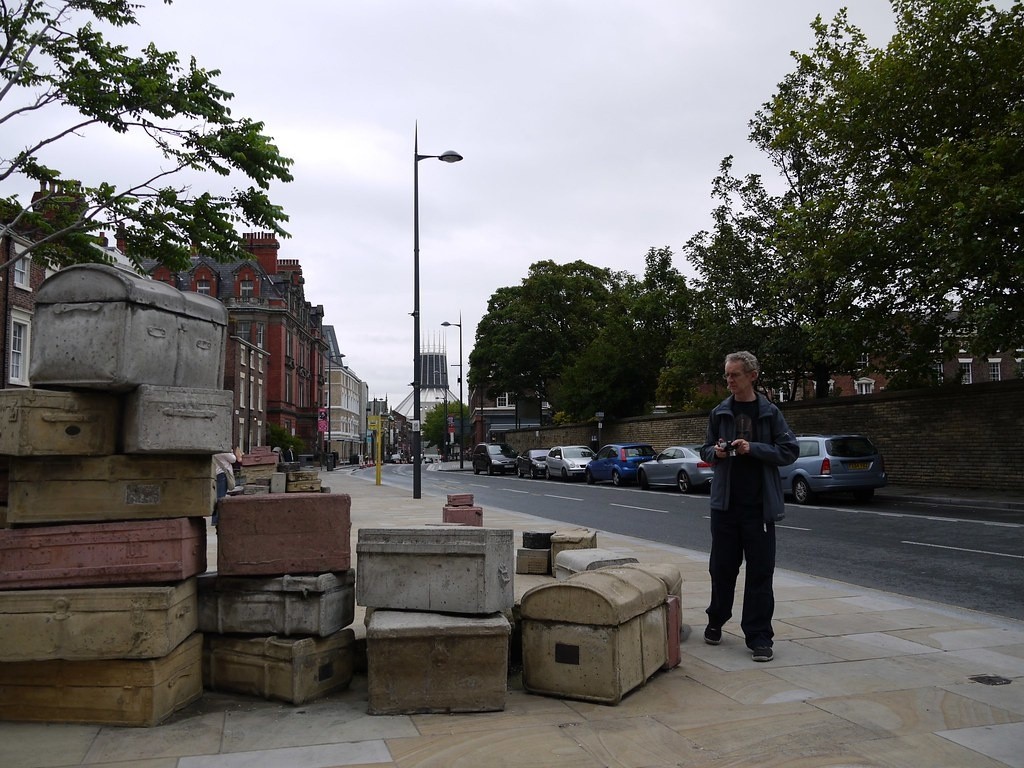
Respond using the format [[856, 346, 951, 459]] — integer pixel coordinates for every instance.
[[327, 454, 334, 471], [350, 455, 359, 464], [298, 454, 314, 467]]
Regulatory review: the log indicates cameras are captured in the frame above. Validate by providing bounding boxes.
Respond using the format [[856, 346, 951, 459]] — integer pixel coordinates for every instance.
[[720, 441, 739, 456]]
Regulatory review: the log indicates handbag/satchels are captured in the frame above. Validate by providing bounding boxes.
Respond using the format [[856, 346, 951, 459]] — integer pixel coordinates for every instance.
[[225, 464, 235, 490]]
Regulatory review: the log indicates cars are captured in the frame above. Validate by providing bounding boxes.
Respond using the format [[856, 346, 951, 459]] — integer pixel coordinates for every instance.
[[409, 455, 434, 464], [514, 448, 551, 480], [545, 446, 598, 482], [636, 444, 715, 494], [584, 442, 659, 488]]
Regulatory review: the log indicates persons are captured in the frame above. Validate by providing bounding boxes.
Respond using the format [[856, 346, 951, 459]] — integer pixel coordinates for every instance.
[[699, 351, 800, 662], [285, 445, 298, 462], [210, 442, 236, 527], [332, 450, 338, 468], [364, 454, 369, 466]]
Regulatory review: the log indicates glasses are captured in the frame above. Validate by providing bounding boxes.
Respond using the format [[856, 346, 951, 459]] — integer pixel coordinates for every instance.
[[723, 371, 750, 379]]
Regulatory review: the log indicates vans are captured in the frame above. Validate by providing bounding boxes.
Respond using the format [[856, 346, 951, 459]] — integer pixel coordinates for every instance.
[[390, 453, 404, 462]]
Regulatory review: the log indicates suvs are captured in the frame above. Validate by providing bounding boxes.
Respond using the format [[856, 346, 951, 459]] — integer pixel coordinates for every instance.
[[472, 442, 521, 476], [775, 433, 887, 505]]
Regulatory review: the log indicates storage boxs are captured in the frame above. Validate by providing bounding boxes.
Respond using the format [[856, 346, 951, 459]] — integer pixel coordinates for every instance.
[[0, 265, 685, 733]]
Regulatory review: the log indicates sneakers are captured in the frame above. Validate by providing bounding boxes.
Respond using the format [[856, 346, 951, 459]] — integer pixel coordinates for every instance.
[[704, 626, 722, 644], [752, 643, 773, 661]]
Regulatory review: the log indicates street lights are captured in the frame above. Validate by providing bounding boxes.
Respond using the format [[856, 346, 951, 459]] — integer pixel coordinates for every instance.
[[435, 397, 447, 462], [411, 117, 464, 499], [328, 354, 346, 453], [440, 309, 463, 468]]
